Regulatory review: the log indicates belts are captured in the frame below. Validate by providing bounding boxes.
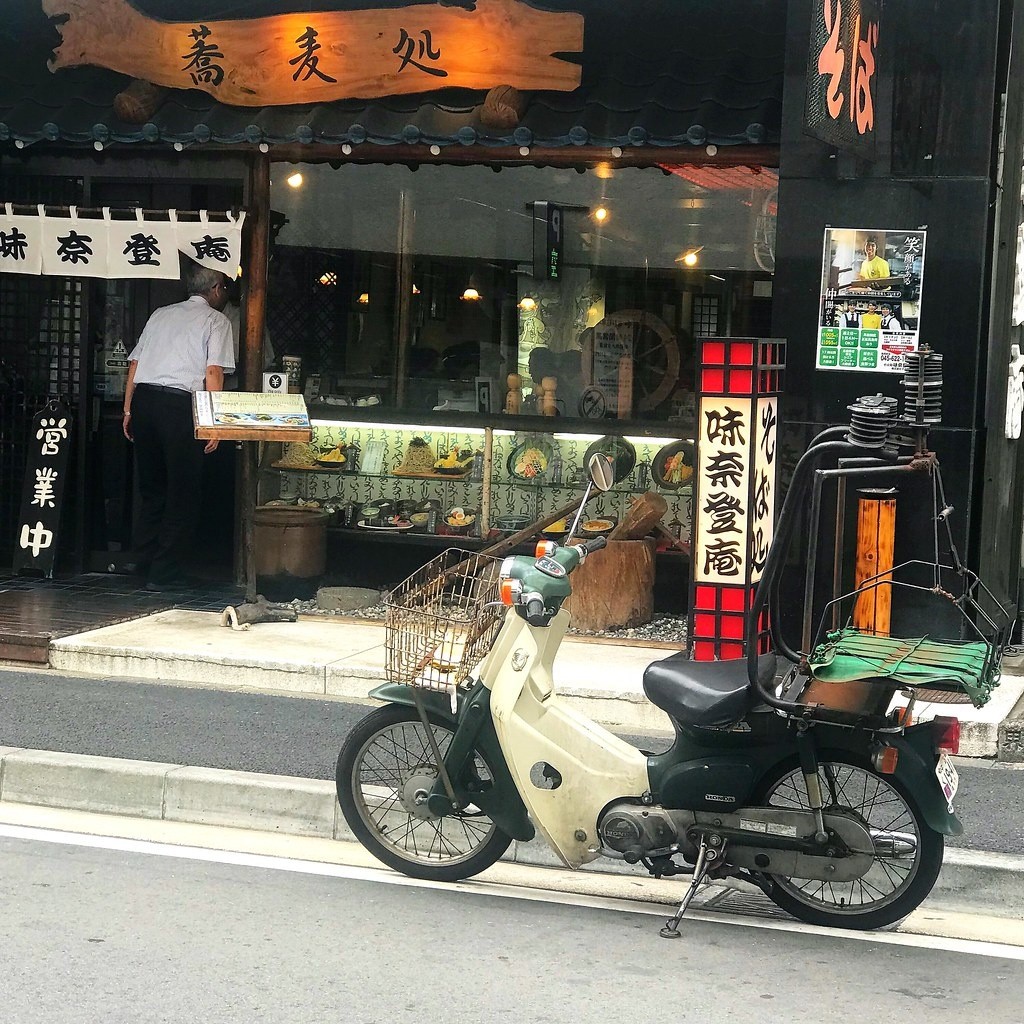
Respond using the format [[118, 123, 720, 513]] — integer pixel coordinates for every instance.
[[137, 383, 192, 399]]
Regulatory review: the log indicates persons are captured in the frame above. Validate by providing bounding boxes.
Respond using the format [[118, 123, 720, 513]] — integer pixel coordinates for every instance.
[[649, 330, 692, 422], [122, 268, 236, 591], [840, 300, 902, 331], [527, 325, 597, 418], [860, 238, 891, 292]]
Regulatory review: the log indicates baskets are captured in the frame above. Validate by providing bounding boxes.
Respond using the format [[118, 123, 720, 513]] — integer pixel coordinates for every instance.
[[382, 548, 512, 696]]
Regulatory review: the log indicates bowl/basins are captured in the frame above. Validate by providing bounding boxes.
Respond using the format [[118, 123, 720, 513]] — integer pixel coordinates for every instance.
[[442, 506, 475, 534], [651, 441, 697, 489], [416, 499, 440, 513], [279, 494, 296, 505], [411, 513, 429, 527], [361, 508, 380, 521], [371, 499, 394, 515], [394, 499, 417, 523], [507, 440, 554, 481], [583, 436, 636, 484], [495, 515, 531, 530]]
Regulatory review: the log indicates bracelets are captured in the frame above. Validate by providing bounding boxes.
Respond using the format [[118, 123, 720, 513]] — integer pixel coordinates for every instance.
[[123, 411, 132, 416]]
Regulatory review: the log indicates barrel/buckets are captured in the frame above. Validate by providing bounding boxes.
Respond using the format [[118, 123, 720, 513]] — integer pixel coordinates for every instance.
[[242, 507, 328, 599]]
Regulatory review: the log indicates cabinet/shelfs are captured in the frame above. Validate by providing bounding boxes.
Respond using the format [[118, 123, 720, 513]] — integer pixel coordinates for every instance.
[[257, 397, 698, 619]]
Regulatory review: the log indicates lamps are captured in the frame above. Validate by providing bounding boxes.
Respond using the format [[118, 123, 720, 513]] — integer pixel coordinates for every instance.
[[459, 286, 484, 302], [517, 290, 538, 311], [355, 292, 370, 306], [412, 282, 421, 294], [319, 271, 338, 286]]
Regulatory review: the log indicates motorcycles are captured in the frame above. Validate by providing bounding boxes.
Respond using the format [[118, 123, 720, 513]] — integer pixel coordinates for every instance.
[[336, 450, 964, 940]]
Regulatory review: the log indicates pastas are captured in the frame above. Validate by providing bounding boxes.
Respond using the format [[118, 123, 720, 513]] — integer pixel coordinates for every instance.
[[395, 438, 437, 473], [278, 441, 317, 466]]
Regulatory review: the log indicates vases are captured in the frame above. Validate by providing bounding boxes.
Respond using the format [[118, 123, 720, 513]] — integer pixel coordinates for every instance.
[[242, 503, 330, 601]]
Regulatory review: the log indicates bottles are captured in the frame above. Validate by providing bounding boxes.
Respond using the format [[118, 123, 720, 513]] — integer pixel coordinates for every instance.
[[554, 451, 562, 481], [638, 462, 647, 488], [428, 509, 436, 533], [345, 501, 352, 526], [475, 508, 482, 536], [345, 446, 354, 471], [471, 453, 482, 479]]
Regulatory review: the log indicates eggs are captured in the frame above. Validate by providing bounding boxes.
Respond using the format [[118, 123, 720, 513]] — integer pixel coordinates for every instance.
[[456, 512, 465, 520], [514, 462, 526, 473]]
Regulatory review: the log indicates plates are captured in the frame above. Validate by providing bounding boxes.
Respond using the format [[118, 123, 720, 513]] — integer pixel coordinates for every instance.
[[264, 499, 286, 506], [581, 519, 614, 533], [358, 521, 414, 529], [542, 522, 570, 533], [432, 464, 468, 474], [316, 458, 347, 468]]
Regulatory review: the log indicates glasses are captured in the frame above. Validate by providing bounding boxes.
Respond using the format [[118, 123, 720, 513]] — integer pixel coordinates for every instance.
[[211, 282, 229, 290]]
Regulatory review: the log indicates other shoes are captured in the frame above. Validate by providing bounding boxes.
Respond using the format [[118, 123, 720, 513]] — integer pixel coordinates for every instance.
[[146, 575, 209, 592]]
